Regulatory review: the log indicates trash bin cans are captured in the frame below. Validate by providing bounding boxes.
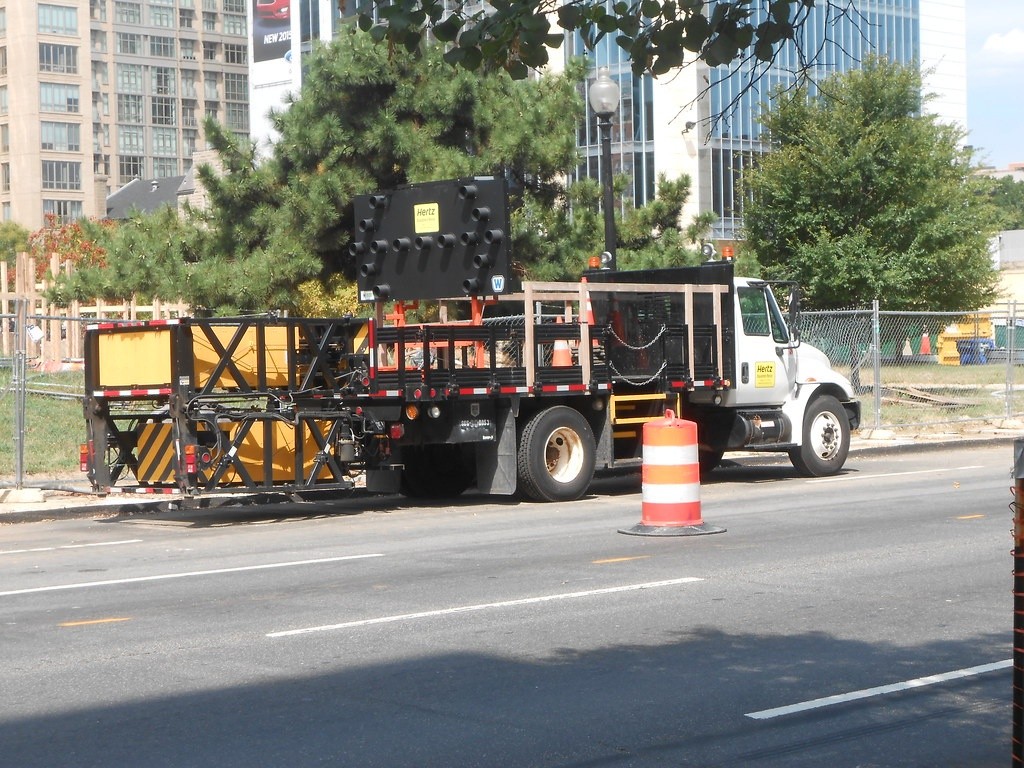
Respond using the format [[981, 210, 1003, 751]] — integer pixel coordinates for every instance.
[[956, 339, 995, 365]]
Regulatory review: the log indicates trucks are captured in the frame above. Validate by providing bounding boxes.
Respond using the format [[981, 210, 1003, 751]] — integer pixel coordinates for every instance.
[[77, 167, 861, 508]]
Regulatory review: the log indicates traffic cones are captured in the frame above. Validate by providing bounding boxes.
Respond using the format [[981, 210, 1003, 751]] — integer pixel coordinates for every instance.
[[551, 309, 575, 367], [574, 275, 600, 347], [919, 326, 932, 355], [608, 292, 628, 352]]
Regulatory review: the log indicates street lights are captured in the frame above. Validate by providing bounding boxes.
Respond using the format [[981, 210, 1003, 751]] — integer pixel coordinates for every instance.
[[587, 68, 621, 271]]
